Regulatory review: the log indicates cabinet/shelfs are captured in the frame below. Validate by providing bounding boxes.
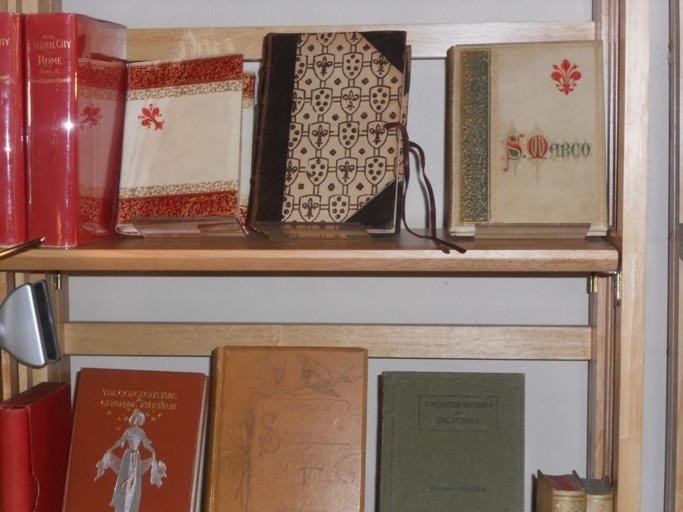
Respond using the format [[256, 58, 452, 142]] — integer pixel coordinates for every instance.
[[0, 1, 647, 512]]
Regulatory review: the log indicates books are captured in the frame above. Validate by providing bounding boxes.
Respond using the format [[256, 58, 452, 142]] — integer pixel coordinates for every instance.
[[535, 470, 615, 512], [379, 370, 524, 511], [445, 40, 610, 238], [62, 368, 210, 512], [205, 346, 368, 512], [115, 53, 253, 238], [3, 10, 129, 248], [250, 32, 411, 237], [0, 383, 71, 512]]
